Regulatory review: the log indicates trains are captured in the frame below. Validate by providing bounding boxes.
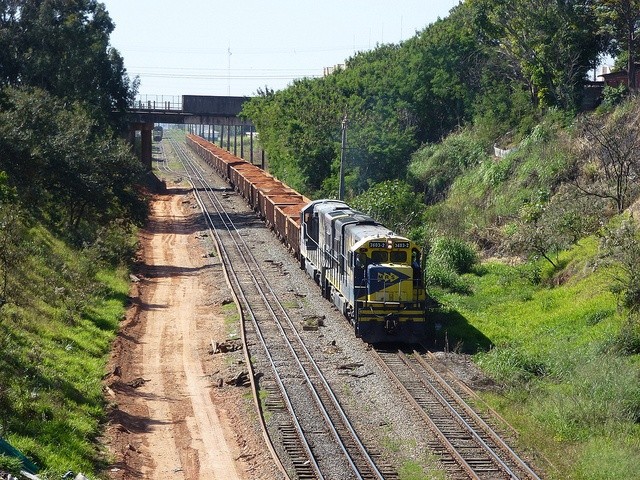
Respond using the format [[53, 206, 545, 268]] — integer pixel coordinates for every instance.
[[185, 134, 429, 342], [153, 126, 163, 141]]
